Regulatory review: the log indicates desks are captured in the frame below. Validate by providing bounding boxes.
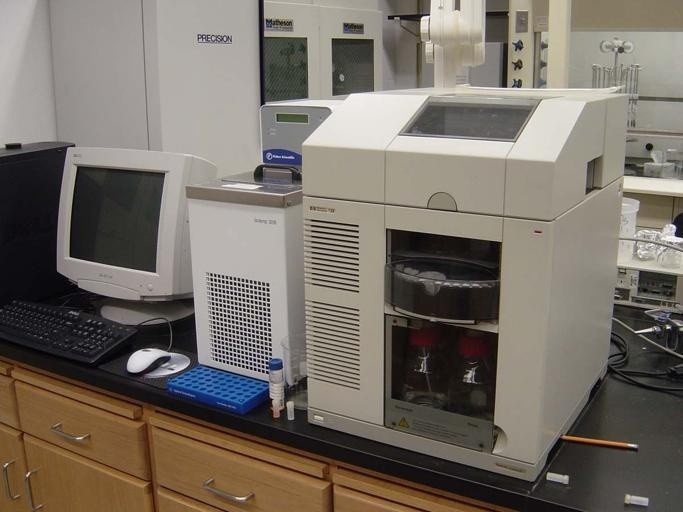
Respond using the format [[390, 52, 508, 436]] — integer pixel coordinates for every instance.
[[621, 175, 683, 233]]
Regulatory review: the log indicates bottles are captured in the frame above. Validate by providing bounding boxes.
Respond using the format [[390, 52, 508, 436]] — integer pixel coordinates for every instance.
[[269, 358, 285, 411], [402, 328, 493, 416]]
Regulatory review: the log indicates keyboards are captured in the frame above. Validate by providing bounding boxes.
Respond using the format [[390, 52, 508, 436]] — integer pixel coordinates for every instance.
[[0, 296, 139, 369]]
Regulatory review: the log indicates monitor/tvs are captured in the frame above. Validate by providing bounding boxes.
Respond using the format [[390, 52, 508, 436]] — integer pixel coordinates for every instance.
[[56, 146, 217, 325]]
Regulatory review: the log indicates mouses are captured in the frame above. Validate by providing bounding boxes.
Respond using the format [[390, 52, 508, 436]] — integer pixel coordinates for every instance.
[[126, 346, 172, 375]]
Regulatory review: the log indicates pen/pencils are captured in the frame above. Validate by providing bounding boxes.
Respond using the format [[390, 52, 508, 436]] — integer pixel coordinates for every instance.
[[560, 435, 639, 450]]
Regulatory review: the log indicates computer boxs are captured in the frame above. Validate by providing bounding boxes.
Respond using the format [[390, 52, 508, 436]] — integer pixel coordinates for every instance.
[[0, 143, 77, 295]]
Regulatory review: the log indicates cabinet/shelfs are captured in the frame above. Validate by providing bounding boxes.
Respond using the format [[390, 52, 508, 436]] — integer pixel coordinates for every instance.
[[0, 362, 517, 512]]
